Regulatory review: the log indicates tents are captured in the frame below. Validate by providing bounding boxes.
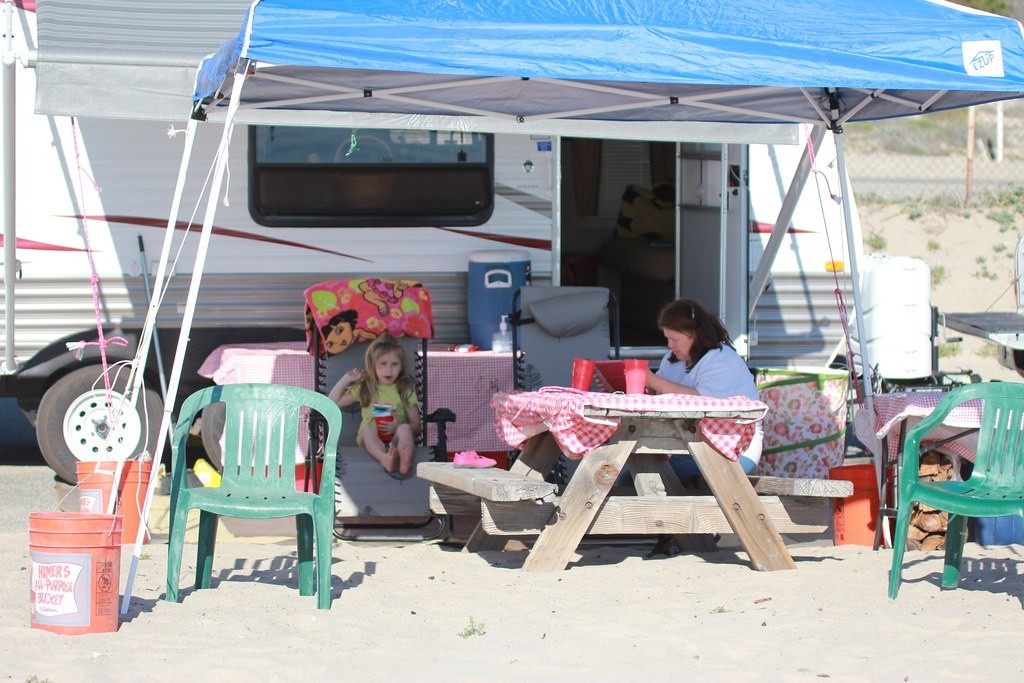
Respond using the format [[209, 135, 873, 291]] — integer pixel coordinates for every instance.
[[106, 1, 1024, 618]]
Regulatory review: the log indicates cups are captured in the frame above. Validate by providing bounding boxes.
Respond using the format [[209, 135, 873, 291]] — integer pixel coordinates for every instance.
[[572, 358, 597, 392], [625, 359, 648, 394], [374, 416, 394, 441]]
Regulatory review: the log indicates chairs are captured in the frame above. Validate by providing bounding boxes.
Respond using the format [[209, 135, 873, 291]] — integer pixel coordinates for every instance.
[[507, 286, 619, 484], [597, 240, 675, 335], [166, 383, 342, 609], [303, 278, 456, 541], [887, 382, 1024, 599]]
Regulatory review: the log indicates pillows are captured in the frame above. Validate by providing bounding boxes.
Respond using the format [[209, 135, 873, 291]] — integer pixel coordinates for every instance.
[[614, 185, 674, 238]]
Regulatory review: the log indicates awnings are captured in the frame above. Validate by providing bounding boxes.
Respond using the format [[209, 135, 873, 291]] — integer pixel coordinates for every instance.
[[2, 0, 833, 370]]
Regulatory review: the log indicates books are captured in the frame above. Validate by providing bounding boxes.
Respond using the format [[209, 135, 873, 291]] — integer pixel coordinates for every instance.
[[595, 360, 649, 394]]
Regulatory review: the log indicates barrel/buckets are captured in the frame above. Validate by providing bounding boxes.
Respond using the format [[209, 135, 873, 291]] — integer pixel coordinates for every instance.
[[75, 461, 152, 544], [830, 465, 893, 546], [466, 249, 534, 352], [29, 513, 124, 635], [846, 252, 937, 378]]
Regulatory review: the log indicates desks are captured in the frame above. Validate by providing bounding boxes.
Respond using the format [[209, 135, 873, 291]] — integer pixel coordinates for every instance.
[[462, 391, 796, 573], [197, 343, 515, 495], [853, 392, 1024, 550]]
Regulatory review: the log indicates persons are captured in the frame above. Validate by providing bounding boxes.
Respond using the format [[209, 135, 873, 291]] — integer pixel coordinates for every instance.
[[327, 333, 423, 475], [592, 299, 763, 556]]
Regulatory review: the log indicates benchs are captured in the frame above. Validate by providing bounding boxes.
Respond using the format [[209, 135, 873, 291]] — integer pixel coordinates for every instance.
[[417, 462, 854, 535]]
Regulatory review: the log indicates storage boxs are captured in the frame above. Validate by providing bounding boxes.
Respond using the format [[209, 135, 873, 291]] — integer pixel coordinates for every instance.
[[467, 249, 532, 351]]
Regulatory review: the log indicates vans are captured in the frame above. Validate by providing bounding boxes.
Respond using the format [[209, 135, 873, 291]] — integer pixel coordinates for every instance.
[[0, 0, 869, 486]]
